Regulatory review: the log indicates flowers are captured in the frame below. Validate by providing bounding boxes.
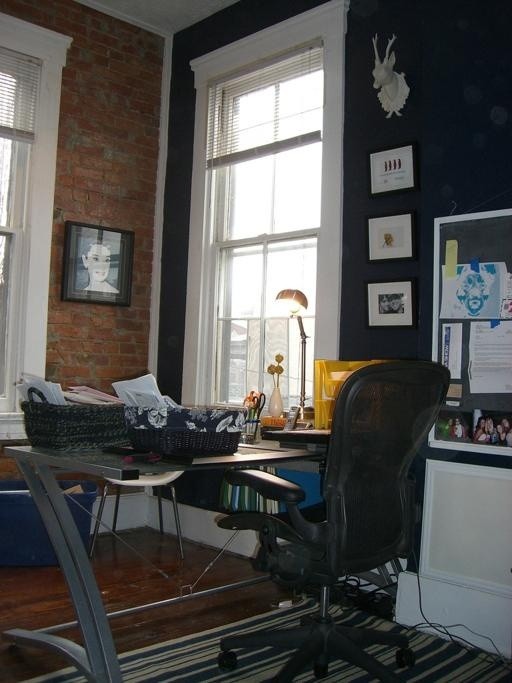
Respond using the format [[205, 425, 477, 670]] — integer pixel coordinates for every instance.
[[265, 353, 289, 386]]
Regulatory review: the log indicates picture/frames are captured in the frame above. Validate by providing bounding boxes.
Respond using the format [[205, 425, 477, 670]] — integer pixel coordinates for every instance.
[[366, 139, 418, 200], [60, 220, 137, 308], [364, 276, 419, 331], [364, 209, 418, 265]]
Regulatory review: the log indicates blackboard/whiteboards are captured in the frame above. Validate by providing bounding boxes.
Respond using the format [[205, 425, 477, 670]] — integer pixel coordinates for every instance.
[[425, 209, 511, 472]]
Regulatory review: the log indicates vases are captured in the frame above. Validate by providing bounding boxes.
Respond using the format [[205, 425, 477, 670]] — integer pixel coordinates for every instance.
[[269, 386, 284, 416]]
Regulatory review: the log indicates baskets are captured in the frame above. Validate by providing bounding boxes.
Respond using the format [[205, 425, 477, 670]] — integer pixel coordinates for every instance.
[[124, 400, 248, 456], [20, 385, 134, 452]]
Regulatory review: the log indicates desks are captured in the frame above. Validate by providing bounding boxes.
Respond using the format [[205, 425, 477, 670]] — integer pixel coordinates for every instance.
[[2, 427, 333, 680]]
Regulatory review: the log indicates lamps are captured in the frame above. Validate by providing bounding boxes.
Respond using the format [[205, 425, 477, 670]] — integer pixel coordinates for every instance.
[[274, 290, 310, 420]]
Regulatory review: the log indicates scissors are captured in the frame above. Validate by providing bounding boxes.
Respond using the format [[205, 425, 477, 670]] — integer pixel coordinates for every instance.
[[243, 393, 265, 432]]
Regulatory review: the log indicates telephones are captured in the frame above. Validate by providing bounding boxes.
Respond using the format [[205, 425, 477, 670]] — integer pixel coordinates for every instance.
[[284, 407, 311, 431]]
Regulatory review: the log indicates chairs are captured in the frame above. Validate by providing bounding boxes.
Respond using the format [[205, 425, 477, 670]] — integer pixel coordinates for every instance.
[[201, 360, 451, 681]]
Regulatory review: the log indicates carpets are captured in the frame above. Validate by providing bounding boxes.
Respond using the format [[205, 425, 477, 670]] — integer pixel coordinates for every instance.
[[21, 595, 512, 681]]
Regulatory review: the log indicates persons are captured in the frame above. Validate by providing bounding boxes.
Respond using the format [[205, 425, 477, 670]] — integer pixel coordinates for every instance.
[[81, 239, 120, 293], [379, 295, 392, 313], [439, 415, 510, 444], [384, 294, 404, 313], [457, 264, 495, 317]]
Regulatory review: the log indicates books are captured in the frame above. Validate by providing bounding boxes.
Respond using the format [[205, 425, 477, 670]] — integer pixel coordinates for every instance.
[[219, 464, 279, 515]]
